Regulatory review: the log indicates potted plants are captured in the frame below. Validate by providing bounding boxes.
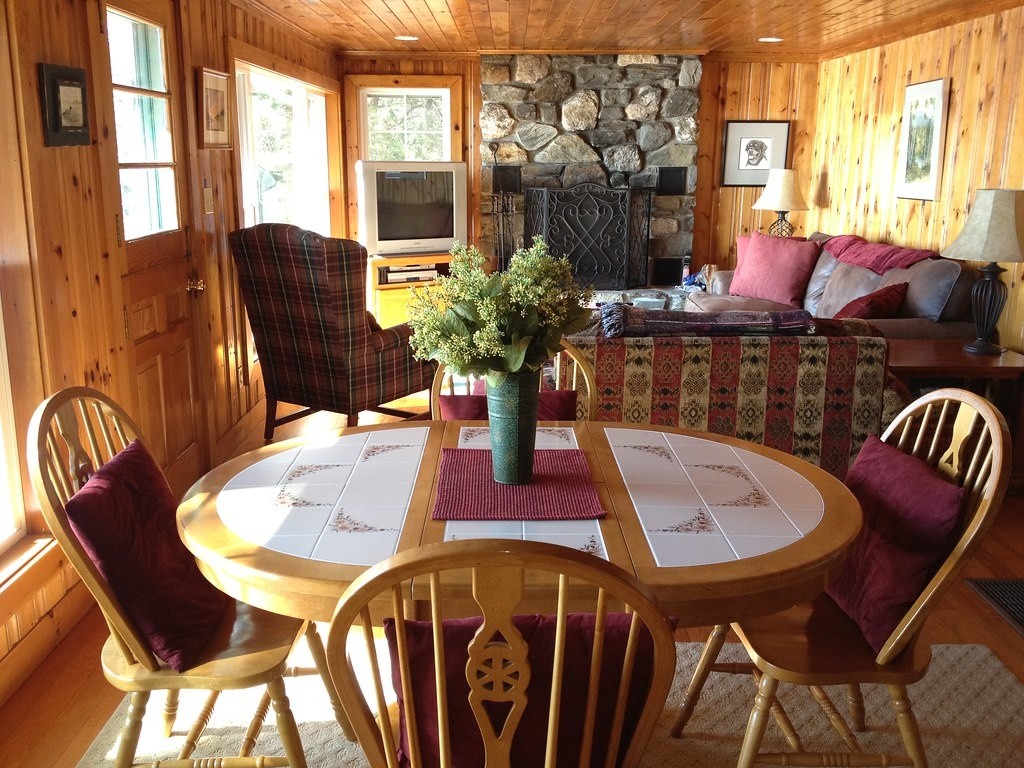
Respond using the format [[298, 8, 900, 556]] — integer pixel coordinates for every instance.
[[408, 245, 598, 486]]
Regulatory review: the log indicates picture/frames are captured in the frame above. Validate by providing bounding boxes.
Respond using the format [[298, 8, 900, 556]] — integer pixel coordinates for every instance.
[[720, 121, 791, 187], [196, 66, 234, 150], [895, 77, 951, 202], [38, 63, 92, 147]]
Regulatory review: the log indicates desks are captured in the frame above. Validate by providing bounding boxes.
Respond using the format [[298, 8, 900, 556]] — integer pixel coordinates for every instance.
[[883, 335, 1024, 490], [174, 421, 861, 768]]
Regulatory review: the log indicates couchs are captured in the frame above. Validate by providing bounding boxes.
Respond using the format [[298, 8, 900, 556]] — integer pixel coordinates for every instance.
[[558, 231, 979, 481]]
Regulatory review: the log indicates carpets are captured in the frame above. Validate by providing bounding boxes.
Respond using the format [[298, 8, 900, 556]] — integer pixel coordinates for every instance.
[[81, 599, 1024, 768]]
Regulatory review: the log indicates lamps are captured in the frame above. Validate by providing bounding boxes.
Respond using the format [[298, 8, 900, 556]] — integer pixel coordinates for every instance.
[[750, 168, 810, 237], [938, 187, 1024, 354]]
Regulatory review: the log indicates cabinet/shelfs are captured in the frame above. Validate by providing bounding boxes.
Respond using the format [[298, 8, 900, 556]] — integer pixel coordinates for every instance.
[[366, 250, 468, 316]]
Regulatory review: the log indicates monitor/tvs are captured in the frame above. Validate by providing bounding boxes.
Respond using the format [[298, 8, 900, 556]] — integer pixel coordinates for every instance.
[[356, 161, 468, 256]]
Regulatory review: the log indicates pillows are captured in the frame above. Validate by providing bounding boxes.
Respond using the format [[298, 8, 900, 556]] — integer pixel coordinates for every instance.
[[728, 232, 823, 307], [832, 282, 909, 317]]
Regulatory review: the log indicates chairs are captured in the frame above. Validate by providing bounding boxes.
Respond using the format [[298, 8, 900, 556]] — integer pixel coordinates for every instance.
[[430, 337, 596, 421], [324, 538, 674, 767], [672, 389, 1013, 768], [227, 224, 440, 441], [26, 387, 358, 768]]
